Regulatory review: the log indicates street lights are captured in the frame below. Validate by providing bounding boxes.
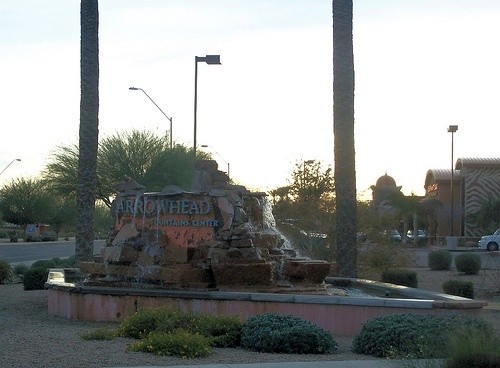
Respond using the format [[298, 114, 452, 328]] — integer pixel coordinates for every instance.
[[129, 87, 173, 150], [201, 145, 230, 179], [447, 125, 465, 237], [193, 55, 221, 159]]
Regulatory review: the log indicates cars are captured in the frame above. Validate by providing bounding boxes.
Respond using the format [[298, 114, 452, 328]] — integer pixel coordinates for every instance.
[[407, 230, 425, 241]]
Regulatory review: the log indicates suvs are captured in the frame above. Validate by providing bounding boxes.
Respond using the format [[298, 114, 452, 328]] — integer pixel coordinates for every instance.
[[383, 230, 401, 243], [478, 226, 500, 251]]
